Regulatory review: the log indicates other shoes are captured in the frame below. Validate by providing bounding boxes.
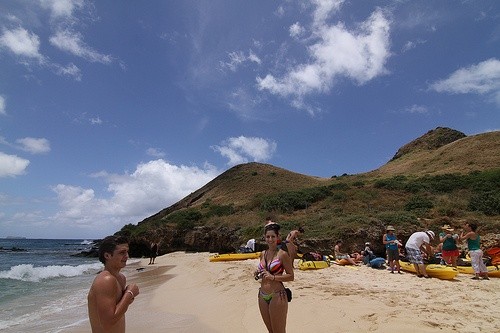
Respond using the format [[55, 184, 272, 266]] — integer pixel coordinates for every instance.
[[398, 272, 402, 274], [425, 274, 432, 278], [390, 271, 394, 273], [471, 277, 479, 280], [483, 277, 490, 280], [417, 274, 423, 277]]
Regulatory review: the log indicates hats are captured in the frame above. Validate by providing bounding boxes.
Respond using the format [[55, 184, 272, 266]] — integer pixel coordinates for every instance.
[[387, 226, 395, 231], [426, 230, 435, 240], [441, 225, 454, 231]]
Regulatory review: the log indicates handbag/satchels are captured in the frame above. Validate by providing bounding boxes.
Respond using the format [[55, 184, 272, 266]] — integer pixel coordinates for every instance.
[[284, 288, 292, 302]]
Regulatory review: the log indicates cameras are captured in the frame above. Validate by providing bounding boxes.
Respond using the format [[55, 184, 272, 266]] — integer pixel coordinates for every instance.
[[255, 272, 264, 280]]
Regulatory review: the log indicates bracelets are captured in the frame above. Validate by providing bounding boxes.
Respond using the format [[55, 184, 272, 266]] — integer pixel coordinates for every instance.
[[273, 275, 276, 280], [126, 289, 134, 297]]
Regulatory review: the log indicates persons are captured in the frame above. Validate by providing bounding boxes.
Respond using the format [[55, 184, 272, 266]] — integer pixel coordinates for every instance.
[[439, 224, 459, 269], [405, 230, 436, 279], [460, 223, 489, 280], [363, 241, 373, 265], [148, 240, 158, 264], [263, 216, 280, 228], [382, 225, 404, 275], [334, 239, 360, 268], [86, 235, 140, 333], [254, 224, 295, 333], [286, 228, 304, 270], [483, 239, 500, 266]]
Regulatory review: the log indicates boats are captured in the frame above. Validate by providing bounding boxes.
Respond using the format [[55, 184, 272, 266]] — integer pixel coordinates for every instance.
[[208, 252, 262, 262], [292, 253, 330, 271], [336, 252, 387, 269], [398, 249, 500, 280]]
